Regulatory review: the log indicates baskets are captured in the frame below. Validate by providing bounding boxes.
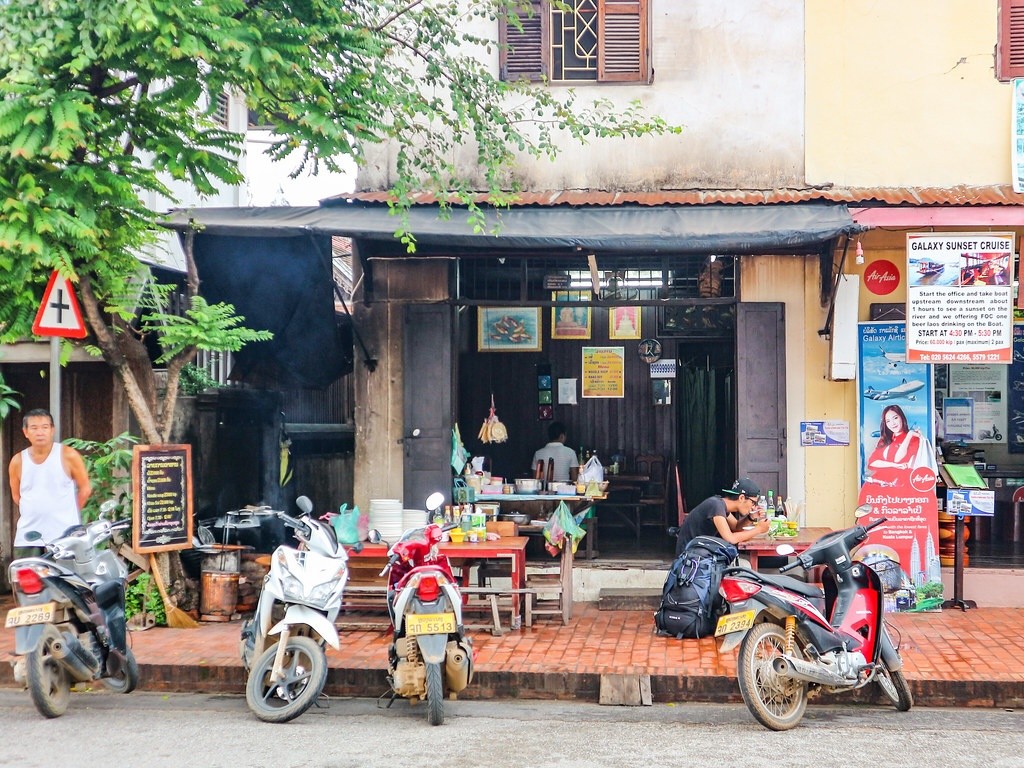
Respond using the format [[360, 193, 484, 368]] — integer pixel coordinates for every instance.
[[868, 559, 902, 593]]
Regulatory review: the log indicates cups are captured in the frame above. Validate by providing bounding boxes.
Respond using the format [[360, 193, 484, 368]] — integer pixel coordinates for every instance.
[[782, 522, 797, 532]]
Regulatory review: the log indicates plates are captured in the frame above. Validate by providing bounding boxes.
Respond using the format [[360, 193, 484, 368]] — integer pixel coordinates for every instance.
[[368, 499, 428, 542], [742, 526, 771, 538], [774, 536, 797, 541]]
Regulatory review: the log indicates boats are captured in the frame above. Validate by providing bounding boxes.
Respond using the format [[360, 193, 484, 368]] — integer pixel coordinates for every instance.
[[916, 260, 945, 274]]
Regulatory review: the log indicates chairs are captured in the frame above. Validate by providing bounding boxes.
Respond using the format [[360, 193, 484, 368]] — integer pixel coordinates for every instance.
[[634, 454, 665, 529], [675, 459, 689, 527], [527, 532, 573, 620], [637, 460, 672, 536], [525, 533, 569, 626]]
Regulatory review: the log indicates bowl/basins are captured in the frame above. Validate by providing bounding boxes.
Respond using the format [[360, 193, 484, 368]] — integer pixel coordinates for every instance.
[[439, 532, 449, 542], [449, 532, 466, 542], [498, 514, 530, 524], [502, 484, 514, 493], [531, 520, 548, 526], [514, 479, 540, 494]]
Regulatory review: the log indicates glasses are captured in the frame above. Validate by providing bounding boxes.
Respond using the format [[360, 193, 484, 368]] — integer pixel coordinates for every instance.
[[744, 496, 757, 506]]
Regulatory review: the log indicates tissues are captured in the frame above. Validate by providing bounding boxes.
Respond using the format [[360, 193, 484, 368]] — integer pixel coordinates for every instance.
[[771, 514, 787, 528]]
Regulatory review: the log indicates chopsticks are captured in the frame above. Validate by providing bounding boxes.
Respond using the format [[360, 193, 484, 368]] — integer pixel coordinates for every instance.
[[784, 499, 805, 522], [765, 518, 768, 523]]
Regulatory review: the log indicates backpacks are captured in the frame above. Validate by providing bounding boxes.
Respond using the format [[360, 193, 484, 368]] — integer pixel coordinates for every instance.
[[653, 536, 738, 639]]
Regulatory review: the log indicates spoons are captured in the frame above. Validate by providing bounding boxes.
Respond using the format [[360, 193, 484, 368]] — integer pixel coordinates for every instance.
[[749, 509, 760, 514]]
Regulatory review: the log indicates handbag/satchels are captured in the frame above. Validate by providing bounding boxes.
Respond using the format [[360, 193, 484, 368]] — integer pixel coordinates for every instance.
[[543, 503, 584, 558], [578, 455, 605, 484]]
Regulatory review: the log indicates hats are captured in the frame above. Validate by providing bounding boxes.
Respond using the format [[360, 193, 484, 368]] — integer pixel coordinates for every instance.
[[722, 477, 760, 495]]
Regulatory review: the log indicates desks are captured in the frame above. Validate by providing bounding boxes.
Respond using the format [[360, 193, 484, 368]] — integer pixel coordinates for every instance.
[[342, 536, 530, 629], [597, 474, 649, 538], [474, 491, 609, 558], [738, 527, 834, 583]]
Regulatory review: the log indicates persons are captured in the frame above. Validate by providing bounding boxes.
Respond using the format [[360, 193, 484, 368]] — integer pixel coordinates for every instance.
[[675, 478, 771, 561], [530, 422, 579, 483], [866, 405, 920, 469], [8, 410, 93, 557]]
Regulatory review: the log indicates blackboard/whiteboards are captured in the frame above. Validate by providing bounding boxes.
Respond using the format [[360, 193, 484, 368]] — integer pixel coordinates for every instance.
[[133, 445, 193, 552]]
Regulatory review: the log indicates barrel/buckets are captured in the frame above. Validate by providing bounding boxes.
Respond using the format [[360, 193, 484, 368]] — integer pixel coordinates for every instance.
[[199, 570, 239, 616]]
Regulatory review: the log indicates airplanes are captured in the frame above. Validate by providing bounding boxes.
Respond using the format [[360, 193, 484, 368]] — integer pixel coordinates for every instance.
[[864, 378, 925, 401]]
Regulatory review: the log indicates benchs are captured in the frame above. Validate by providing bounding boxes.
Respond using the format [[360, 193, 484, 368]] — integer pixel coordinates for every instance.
[[333, 586, 535, 636]]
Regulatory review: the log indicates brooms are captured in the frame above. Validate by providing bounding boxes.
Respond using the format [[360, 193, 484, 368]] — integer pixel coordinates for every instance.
[[149, 552, 199, 630]]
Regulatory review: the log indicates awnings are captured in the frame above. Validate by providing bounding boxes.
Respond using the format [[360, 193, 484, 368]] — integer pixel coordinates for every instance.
[[155, 202, 1024, 252]]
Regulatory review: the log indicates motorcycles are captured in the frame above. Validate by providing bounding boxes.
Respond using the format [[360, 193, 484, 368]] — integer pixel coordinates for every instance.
[[714, 504, 914, 731], [367, 491, 478, 725], [4, 499, 139, 719], [239, 493, 363, 723]]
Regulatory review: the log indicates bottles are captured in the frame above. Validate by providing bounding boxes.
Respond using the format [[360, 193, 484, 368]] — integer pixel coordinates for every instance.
[[433, 506, 486, 542], [489, 477, 503, 485], [464, 463, 481, 494], [576, 465, 586, 496], [757, 490, 784, 521], [578, 447, 597, 465]]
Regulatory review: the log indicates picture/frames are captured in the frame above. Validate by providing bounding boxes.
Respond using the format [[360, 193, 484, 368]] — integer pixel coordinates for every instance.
[[477, 306, 542, 352], [551, 291, 591, 339], [656, 286, 734, 338], [609, 299, 641, 340]]
